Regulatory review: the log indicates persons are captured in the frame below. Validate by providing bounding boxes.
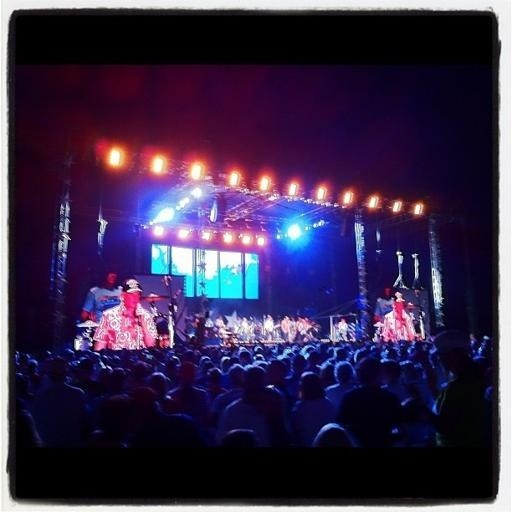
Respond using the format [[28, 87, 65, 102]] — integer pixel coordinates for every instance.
[[9, 269, 498, 502]]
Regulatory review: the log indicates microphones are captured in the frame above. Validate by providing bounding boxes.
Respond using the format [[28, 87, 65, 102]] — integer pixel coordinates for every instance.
[[135, 302, 143, 326]]
